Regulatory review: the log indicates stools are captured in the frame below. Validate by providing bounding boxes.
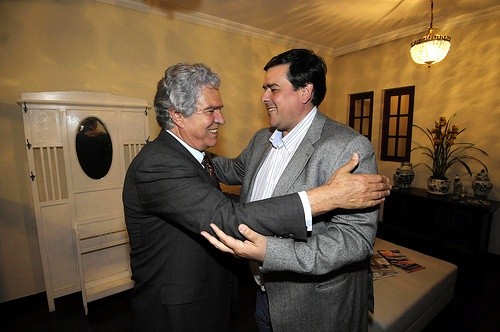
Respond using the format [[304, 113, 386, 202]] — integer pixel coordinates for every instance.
[[368, 237, 458, 332]]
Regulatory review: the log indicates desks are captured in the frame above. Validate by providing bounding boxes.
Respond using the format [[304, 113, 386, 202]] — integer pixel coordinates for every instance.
[[383, 186, 500, 272]]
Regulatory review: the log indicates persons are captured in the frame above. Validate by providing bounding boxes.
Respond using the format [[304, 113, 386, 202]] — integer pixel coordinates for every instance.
[[200, 49, 382, 332], [122, 63, 392, 332]]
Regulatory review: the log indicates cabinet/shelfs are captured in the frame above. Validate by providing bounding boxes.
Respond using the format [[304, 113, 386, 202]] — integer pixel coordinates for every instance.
[[16, 91, 151, 315]]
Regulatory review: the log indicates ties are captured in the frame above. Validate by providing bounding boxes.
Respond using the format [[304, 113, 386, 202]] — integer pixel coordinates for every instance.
[[202, 157, 214, 177]]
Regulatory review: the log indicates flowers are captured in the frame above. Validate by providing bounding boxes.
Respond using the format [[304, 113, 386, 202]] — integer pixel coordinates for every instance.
[[407, 113, 489, 179]]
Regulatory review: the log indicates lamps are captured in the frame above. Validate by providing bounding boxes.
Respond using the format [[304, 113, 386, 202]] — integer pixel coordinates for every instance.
[[410, 0, 451, 68]]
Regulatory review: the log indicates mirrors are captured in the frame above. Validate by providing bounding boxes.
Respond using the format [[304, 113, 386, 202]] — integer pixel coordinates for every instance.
[[76, 117, 113, 179]]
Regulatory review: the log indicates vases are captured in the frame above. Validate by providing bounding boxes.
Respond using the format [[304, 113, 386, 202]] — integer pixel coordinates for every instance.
[[427, 176, 452, 197]]
